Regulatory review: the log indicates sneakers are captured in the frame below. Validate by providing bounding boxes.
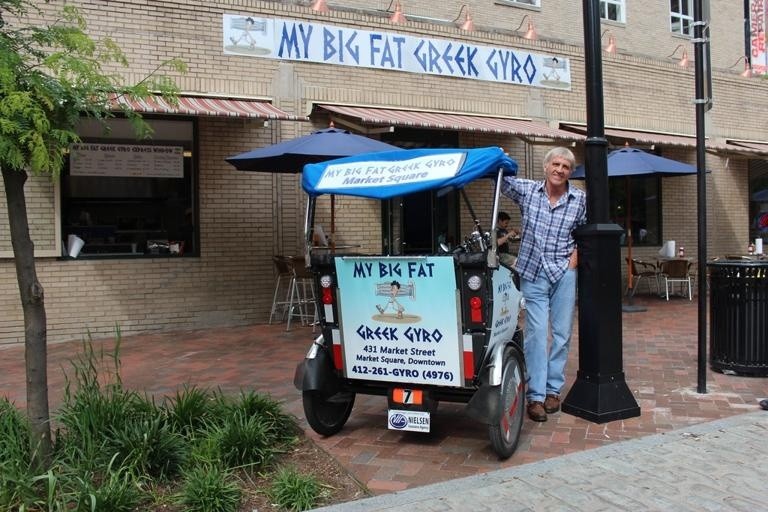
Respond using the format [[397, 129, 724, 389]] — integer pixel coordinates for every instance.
[[544, 394, 560, 413], [526, 401, 547, 421]]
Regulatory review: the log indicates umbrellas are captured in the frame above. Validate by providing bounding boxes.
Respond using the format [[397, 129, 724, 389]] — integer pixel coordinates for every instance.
[[567, 141, 712, 295], [223, 120, 406, 254]]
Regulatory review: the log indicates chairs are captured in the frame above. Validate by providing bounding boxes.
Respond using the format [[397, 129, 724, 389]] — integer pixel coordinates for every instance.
[[266, 252, 318, 333], [625, 252, 766, 301]]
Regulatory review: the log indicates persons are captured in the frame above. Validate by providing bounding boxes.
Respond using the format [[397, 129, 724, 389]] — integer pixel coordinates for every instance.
[[498, 211, 519, 273], [491, 145, 587, 424]]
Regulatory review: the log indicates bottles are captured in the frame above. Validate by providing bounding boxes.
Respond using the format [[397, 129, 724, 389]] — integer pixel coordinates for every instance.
[[679, 247, 684, 258], [324, 235, 329, 244], [748, 242, 754, 254]]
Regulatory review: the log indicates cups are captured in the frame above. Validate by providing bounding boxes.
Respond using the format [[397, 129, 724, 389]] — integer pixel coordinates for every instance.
[[131, 243, 138, 253]]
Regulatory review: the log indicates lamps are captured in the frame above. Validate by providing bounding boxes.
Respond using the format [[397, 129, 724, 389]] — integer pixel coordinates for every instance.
[[515, 15, 539, 41], [452, 3, 474, 32], [386, 1, 408, 24], [668, 44, 691, 68], [310, 1, 330, 13], [600, 28, 616, 56], [730, 55, 755, 80]]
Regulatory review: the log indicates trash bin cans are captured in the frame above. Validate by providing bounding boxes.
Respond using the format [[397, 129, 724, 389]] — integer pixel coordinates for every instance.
[[706, 259, 768, 377]]
